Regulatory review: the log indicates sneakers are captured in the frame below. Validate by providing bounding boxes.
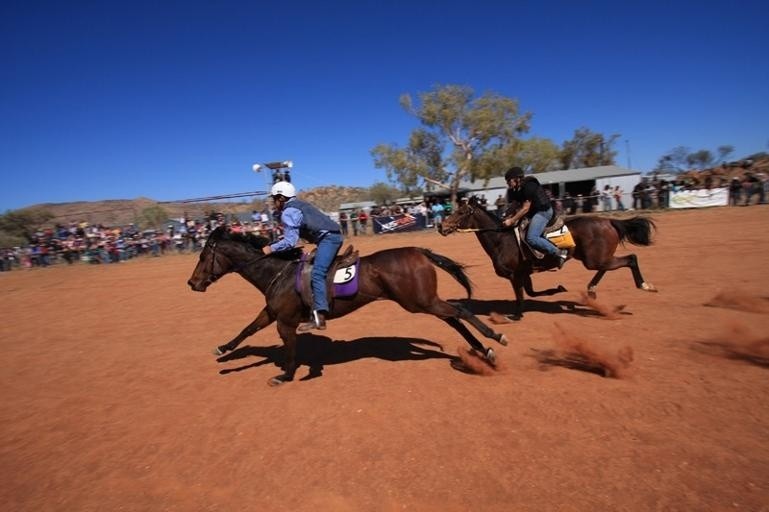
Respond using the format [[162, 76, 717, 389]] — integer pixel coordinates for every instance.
[[555, 248, 567, 268], [298, 312, 326, 331]]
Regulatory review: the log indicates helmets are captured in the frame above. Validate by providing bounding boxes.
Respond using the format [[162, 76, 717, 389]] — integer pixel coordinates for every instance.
[[505, 167, 524, 181], [268, 182, 296, 197]]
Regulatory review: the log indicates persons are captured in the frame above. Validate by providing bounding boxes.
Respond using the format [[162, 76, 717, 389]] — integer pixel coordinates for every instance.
[[502, 167, 568, 271], [629, 159, 769, 207], [334, 192, 509, 238], [545, 183, 628, 216], [261, 180, 344, 331], [0, 199, 283, 271]]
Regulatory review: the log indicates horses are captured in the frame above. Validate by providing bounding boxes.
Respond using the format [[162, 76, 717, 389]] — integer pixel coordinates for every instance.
[[437, 194, 661, 324], [186, 222, 511, 389]]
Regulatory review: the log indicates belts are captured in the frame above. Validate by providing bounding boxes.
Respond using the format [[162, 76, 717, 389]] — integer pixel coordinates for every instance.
[[319, 231, 335, 241]]
